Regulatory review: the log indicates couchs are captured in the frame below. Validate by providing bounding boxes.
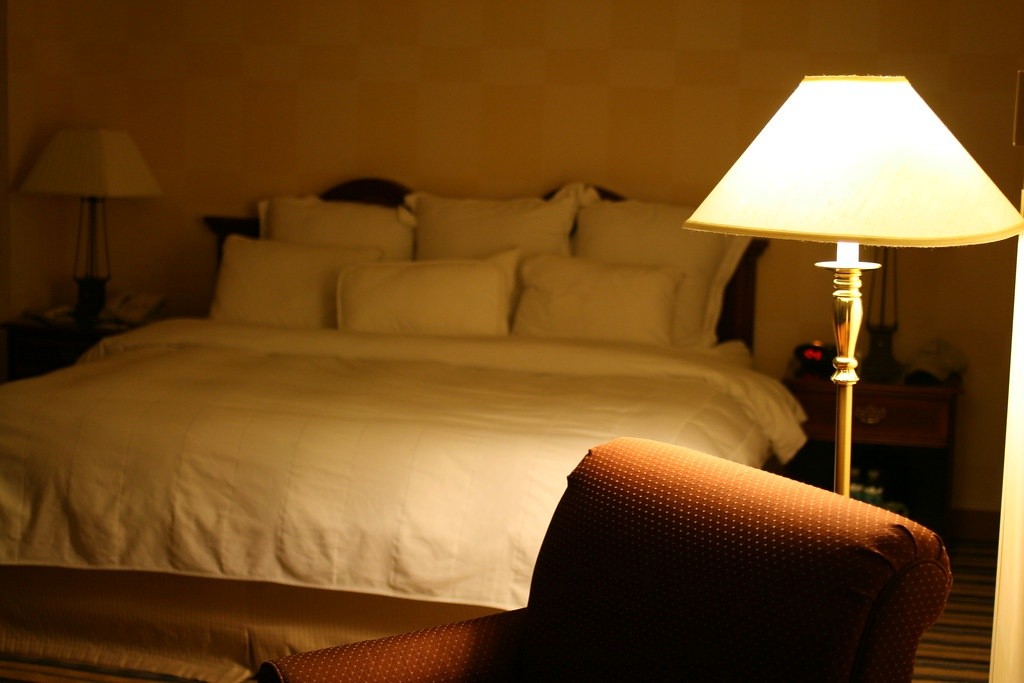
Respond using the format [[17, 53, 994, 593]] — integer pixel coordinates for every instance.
[[259, 438, 954, 683]]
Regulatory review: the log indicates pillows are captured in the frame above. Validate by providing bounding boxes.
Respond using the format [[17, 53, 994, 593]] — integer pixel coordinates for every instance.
[[257, 196, 416, 263], [335, 246, 525, 338], [405, 179, 583, 260], [513, 253, 687, 350], [210, 235, 384, 327], [577, 182, 754, 349]]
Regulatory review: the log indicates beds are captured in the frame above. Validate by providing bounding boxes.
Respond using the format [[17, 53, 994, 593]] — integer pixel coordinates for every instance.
[[0, 179, 810, 683]]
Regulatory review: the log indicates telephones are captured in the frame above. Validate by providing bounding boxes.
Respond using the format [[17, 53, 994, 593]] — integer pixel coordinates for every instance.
[[107, 287, 163, 326]]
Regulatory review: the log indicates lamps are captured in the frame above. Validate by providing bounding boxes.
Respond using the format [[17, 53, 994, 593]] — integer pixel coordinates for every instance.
[[21, 127, 163, 317], [862, 246, 904, 373], [683, 74, 1024, 496]]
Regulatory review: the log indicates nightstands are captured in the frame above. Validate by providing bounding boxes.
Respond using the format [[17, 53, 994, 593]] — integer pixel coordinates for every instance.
[[1, 310, 148, 386], [786, 370, 958, 542]]
